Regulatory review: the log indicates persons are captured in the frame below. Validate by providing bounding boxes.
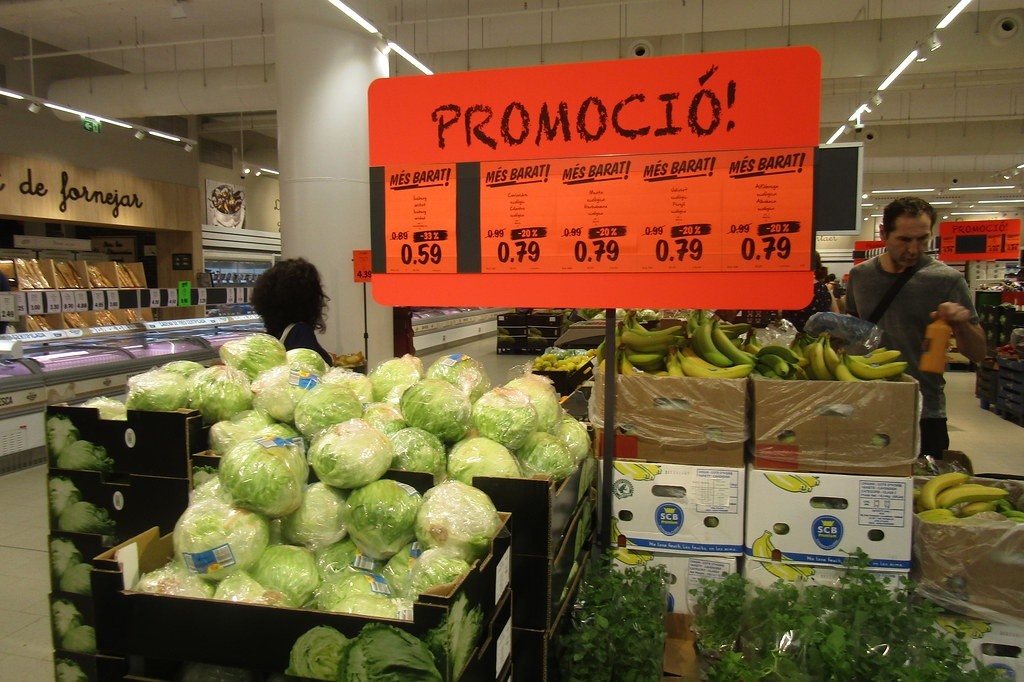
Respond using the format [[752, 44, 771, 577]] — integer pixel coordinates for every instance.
[[845, 195, 986, 460], [782, 251, 849, 331], [251, 257, 332, 368]]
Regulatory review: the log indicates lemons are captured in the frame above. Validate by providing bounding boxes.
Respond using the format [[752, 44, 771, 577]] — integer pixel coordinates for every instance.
[[530, 349, 597, 371]]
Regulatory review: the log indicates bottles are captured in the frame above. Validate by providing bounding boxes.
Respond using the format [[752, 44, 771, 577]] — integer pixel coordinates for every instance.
[[918, 317, 950, 373]]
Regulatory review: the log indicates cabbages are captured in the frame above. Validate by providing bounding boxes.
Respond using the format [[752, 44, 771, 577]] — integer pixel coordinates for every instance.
[[80, 335, 539, 619], [287, 593, 483, 682], [46, 417, 115, 682], [513, 431, 574, 480], [501, 376, 561, 433], [553, 415, 590, 463]]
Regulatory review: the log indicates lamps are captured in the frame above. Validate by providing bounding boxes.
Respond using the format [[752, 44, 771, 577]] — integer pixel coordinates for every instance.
[[242, 165, 250, 174], [254, 169, 261, 177], [927, 30, 941, 51], [170, 0, 186, 18], [184, 143, 194, 152], [843, 121, 853, 136], [914, 43, 927, 63], [872, 90, 883, 105], [135, 130, 146, 140], [375, 37, 392, 56], [28, 103, 42, 113]]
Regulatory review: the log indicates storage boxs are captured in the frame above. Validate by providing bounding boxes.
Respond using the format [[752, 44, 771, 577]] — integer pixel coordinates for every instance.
[[533, 257, 1024, 682], [44, 402, 597, 682], [495, 313, 565, 353]]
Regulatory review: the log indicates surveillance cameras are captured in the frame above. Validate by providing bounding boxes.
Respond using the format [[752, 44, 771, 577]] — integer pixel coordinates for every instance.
[[854, 124, 864, 134]]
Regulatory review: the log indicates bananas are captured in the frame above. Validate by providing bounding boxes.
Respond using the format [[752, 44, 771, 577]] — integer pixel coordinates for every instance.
[[594, 310, 911, 385], [911, 470, 1024, 525]]
